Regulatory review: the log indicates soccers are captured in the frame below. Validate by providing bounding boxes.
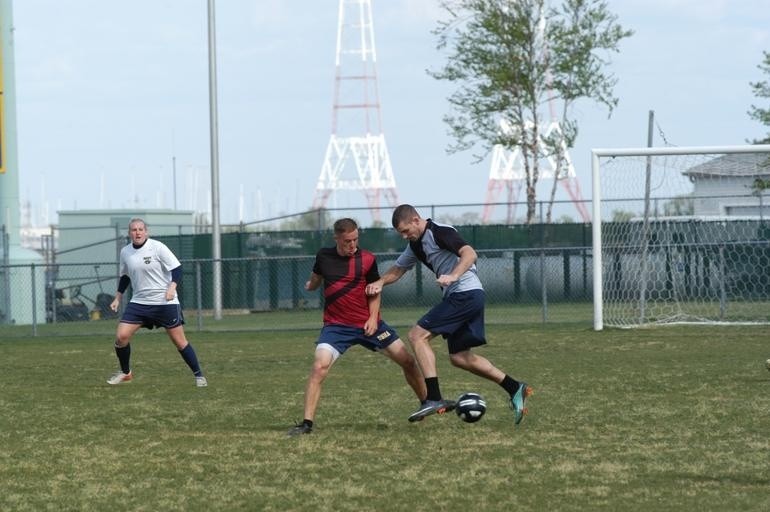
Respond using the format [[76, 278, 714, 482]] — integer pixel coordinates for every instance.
[[457, 393, 485, 423]]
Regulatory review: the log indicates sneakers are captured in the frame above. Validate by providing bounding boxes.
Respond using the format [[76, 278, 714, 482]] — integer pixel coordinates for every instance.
[[106, 369, 133, 385], [510, 383, 533, 425], [407, 398, 459, 422], [195, 377, 208, 387], [288, 421, 312, 436]]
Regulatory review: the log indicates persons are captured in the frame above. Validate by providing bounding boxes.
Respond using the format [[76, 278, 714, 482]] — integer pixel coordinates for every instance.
[[362, 202, 534, 424], [289, 217, 456, 437], [103, 217, 209, 389]]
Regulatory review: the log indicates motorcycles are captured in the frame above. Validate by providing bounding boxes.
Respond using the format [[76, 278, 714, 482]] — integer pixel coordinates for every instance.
[[48, 285, 118, 324]]
